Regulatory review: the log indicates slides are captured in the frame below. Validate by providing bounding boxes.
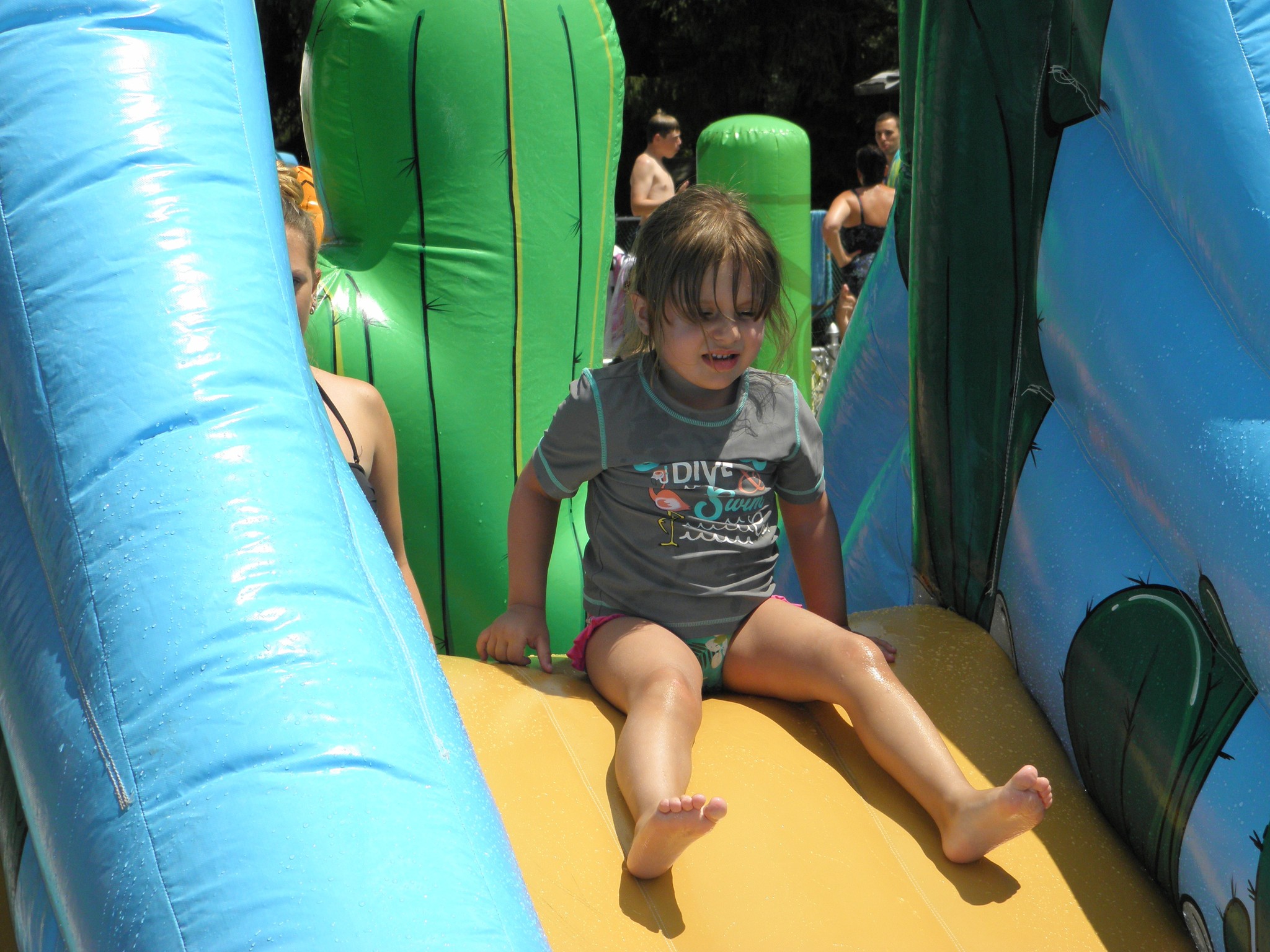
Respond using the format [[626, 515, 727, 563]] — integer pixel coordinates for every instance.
[[0, 1, 1270, 952]]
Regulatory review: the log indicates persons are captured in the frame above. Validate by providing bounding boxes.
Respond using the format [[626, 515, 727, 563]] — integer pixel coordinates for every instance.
[[475, 178, 1054, 880], [275, 161, 439, 659], [822, 144, 896, 342], [629, 114, 689, 225], [875, 113, 902, 189]]
